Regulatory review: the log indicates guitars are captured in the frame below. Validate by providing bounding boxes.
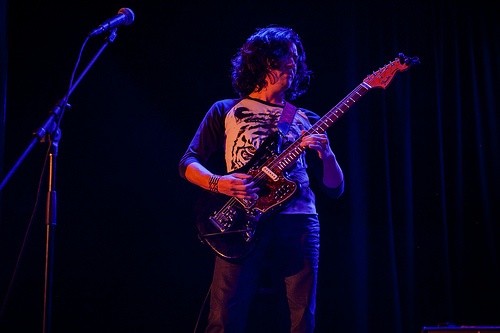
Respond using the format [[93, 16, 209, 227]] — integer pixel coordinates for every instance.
[[190, 54, 410, 261]]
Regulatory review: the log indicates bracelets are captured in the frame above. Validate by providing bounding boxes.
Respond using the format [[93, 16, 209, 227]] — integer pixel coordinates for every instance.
[[208, 174, 221, 192]]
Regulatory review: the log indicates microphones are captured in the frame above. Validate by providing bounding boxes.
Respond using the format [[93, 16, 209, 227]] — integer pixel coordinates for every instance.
[[89, 7, 135, 36]]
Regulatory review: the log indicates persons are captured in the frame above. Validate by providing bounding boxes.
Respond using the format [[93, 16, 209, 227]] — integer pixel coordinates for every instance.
[[180, 27, 344, 333]]
